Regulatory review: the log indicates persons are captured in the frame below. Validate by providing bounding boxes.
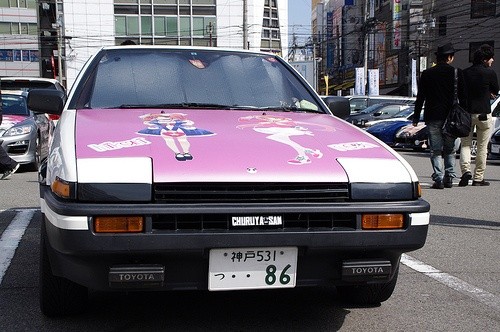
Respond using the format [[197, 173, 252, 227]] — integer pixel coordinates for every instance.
[[407, 43, 497, 190], [0, 78, 20, 180], [456, 45, 500, 186]]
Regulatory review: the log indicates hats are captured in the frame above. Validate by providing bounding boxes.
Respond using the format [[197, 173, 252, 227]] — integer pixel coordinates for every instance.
[[434, 42, 461, 56]]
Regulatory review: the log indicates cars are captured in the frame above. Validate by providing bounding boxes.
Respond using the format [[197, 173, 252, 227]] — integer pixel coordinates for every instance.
[[488, 130, 500, 156], [0, 89, 54, 165], [366, 108, 425, 147], [347, 103, 410, 128], [395, 122, 430, 151], [28, 45, 431, 317]]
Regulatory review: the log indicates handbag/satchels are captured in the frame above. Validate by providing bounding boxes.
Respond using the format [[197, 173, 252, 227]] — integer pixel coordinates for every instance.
[[445, 103, 472, 139]]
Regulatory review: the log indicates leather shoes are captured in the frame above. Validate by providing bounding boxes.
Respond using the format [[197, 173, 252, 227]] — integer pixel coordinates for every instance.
[[432, 177, 452, 189], [472, 179, 490, 186], [458, 171, 472, 187]]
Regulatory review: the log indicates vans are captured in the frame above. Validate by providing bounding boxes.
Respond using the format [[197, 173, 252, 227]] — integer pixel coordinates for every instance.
[[347, 95, 418, 118], [470, 96, 500, 159], [2, 78, 66, 129]]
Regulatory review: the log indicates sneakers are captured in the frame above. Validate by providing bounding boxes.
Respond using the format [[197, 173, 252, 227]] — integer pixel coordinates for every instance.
[[0, 163, 20, 180]]
